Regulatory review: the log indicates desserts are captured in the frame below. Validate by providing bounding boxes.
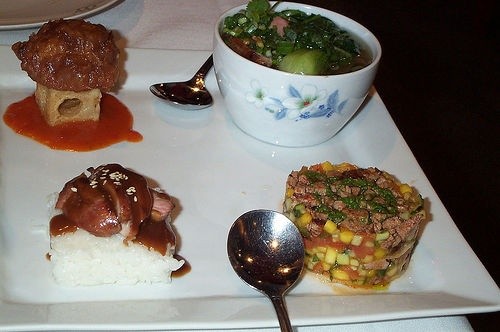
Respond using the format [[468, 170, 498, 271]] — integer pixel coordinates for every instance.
[[3, 18, 143, 152]]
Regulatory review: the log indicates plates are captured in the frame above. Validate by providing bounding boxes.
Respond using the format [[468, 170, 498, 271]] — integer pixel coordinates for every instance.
[[0, 45, 500, 332], [0, 0, 120, 29]]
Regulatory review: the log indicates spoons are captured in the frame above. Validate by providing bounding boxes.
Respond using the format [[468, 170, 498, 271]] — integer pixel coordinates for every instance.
[[149, 55, 213, 110], [228, 211, 305, 332]]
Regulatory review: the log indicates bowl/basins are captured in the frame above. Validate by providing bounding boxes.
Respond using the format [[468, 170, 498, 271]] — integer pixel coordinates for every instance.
[[213, 2, 382, 149]]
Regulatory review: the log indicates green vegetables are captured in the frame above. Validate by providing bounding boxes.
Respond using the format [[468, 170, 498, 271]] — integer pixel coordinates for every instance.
[[220, 0, 359, 71]]
